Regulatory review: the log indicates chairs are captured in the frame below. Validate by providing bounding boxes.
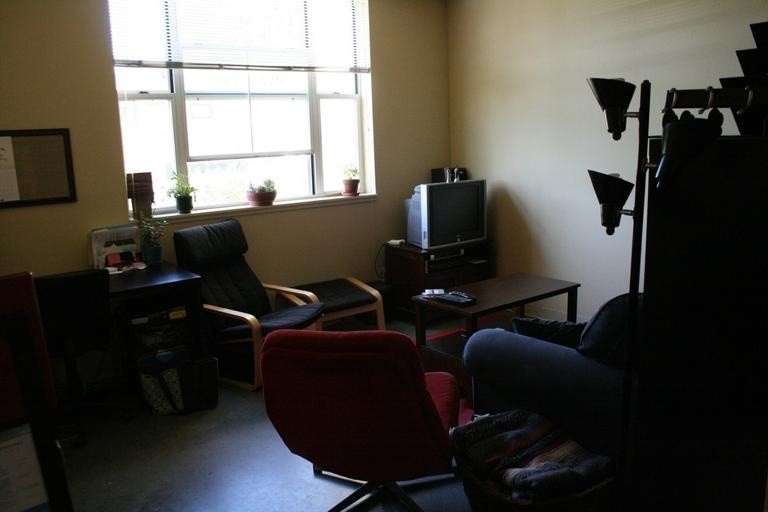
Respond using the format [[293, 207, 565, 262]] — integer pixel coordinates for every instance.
[[255, 328, 462, 512], [455, 297, 716, 511], [176, 222, 321, 396]]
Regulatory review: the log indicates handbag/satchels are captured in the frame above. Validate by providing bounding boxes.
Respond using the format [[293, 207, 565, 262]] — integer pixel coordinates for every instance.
[[136, 357, 185, 417]]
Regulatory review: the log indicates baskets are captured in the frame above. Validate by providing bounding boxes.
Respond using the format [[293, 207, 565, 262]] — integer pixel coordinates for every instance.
[[449, 409, 620, 512]]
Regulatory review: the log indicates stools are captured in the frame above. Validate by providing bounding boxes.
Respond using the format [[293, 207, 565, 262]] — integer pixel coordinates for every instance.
[[275, 275, 383, 330]]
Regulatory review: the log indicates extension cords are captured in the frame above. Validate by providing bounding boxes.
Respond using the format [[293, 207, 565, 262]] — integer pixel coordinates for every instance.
[[388, 239, 406, 245]]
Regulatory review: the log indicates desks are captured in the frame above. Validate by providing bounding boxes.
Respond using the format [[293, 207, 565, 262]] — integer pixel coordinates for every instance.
[[411, 269, 582, 342]]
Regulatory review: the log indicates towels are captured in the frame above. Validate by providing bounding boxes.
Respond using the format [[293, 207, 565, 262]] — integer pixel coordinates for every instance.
[[448, 406, 608, 498]]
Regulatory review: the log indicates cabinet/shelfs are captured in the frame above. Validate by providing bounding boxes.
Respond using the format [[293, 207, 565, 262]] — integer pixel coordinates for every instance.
[[646, 134, 767, 509], [105, 263, 213, 418], [384, 237, 494, 325]]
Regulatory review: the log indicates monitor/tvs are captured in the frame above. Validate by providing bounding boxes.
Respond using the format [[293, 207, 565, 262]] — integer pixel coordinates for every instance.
[[404, 179, 488, 251]]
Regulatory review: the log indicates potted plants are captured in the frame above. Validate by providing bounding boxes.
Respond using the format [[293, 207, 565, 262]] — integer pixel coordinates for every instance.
[[245, 179, 275, 208], [167, 170, 198, 213], [136, 217, 167, 269], [336, 165, 363, 196]]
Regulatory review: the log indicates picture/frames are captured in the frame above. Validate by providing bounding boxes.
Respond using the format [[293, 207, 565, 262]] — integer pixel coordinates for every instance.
[[0, 130, 79, 209]]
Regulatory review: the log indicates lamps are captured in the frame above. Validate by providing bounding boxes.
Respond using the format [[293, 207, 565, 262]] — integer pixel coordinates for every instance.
[[582, 70, 655, 293]]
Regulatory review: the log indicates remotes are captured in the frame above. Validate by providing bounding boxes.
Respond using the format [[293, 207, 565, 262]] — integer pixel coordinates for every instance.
[[449, 290, 475, 299], [436, 294, 473, 307]]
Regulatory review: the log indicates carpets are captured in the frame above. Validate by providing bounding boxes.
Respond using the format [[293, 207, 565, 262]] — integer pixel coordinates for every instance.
[[404, 313, 524, 346]]
